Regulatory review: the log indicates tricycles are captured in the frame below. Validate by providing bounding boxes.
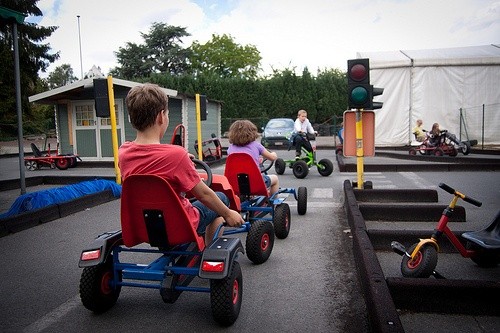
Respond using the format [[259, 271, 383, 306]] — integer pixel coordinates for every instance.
[[391, 183, 500, 279]]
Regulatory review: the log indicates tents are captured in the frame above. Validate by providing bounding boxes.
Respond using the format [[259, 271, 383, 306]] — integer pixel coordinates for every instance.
[[358, 43, 500, 148]]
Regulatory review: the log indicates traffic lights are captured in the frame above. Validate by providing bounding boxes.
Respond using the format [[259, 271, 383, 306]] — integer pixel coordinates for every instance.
[[92, 78, 110, 118], [369, 84, 384, 111], [199, 95, 208, 121], [347, 57, 372, 109]]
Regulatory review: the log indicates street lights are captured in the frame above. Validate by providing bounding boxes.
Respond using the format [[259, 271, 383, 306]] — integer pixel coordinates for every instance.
[[76, 15, 83, 80]]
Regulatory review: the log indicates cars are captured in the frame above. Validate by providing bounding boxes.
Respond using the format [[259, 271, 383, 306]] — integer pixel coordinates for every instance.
[[260, 117, 295, 149]]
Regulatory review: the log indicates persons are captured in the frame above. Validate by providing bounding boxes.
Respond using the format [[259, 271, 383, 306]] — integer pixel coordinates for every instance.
[[117, 83, 245, 245], [431, 123, 443, 146], [338, 122, 344, 144], [291, 109, 316, 171], [228, 120, 279, 200], [413, 119, 430, 146]]
